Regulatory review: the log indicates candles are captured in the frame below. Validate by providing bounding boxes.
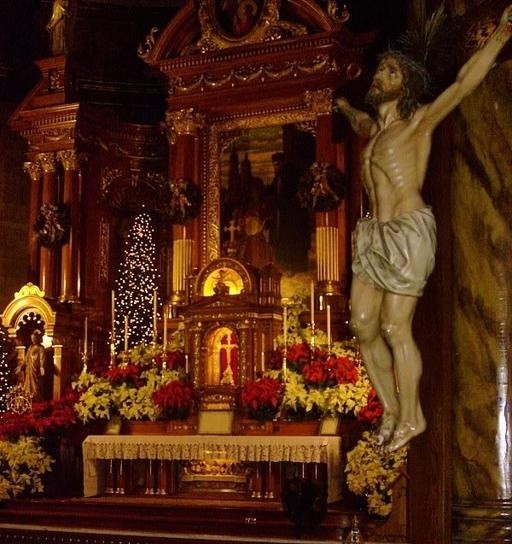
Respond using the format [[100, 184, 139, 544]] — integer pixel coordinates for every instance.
[[281, 278, 332, 347], [82, 287, 169, 353]]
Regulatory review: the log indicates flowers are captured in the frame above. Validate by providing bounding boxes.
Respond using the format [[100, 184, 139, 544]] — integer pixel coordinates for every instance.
[[230, 325, 386, 428], [0, 431, 56, 502], [68, 339, 194, 424], [347, 432, 413, 522]]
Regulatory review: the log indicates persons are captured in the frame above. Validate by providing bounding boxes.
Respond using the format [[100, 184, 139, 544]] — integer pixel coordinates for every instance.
[[254, 152, 311, 272], [14, 329, 48, 400], [333, 4, 512, 451]]
